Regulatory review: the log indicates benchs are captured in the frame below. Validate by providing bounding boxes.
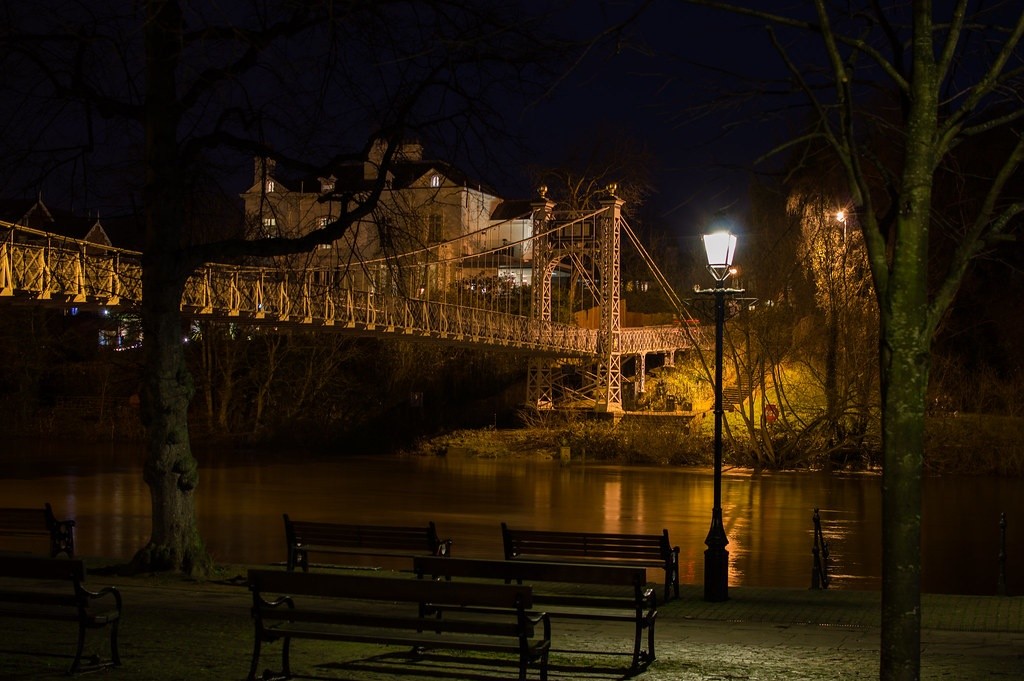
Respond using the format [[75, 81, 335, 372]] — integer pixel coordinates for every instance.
[[0, 503, 122, 676], [247, 513, 680, 681]]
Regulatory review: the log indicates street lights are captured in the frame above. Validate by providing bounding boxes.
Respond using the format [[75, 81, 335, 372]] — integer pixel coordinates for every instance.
[[694, 224, 745, 604]]
